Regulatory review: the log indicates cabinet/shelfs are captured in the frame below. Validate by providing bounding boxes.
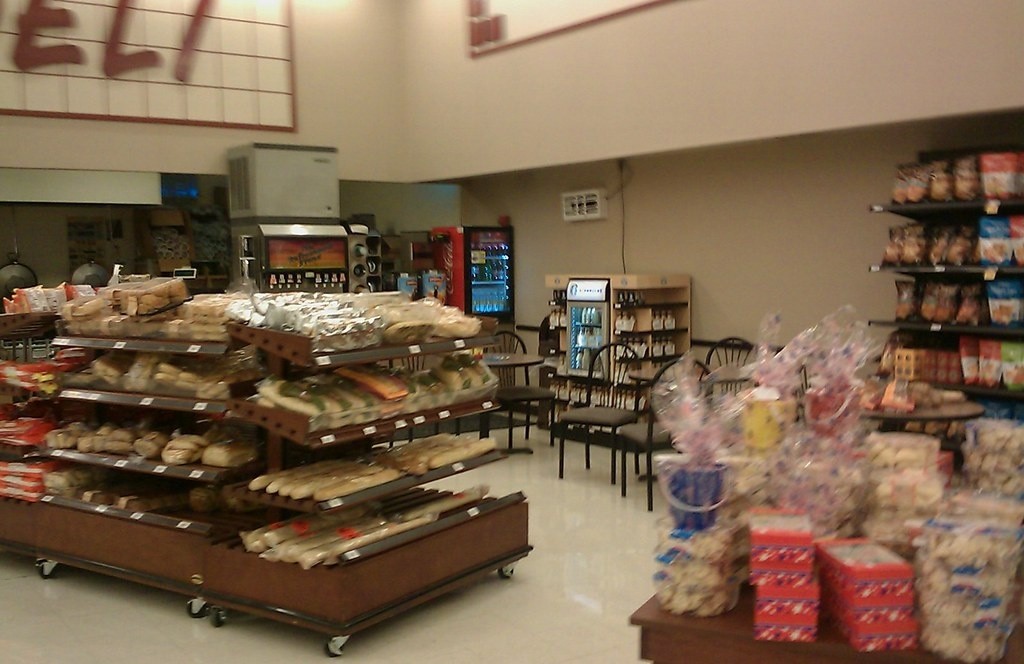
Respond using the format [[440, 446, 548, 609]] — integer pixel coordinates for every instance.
[[544, 273, 691, 455], [867, 143, 1024, 471], [0, 311, 534, 657]]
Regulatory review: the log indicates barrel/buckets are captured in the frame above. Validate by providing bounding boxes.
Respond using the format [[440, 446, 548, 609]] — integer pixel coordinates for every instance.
[[658, 460, 732, 530]]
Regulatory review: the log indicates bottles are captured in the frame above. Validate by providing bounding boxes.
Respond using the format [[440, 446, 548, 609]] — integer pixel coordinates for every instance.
[[616, 309, 676, 357], [471, 242, 508, 312], [549, 308, 566, 327], [549, 380, 649, 412], [575, 307, 601, 371]]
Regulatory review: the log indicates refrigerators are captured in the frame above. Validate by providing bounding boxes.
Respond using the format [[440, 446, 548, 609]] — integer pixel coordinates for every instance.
[[432, 225, 514, 324]]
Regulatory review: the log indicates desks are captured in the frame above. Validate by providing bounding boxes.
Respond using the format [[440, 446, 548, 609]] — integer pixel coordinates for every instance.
[[860, 401, 984, 433], [479, 353, 544, 440], [629, 370, 750, 482], [628, 560, 1024, 664]]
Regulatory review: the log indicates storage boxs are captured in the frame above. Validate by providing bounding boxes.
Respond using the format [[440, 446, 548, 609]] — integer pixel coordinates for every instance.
[[747, 507, 925, 654]]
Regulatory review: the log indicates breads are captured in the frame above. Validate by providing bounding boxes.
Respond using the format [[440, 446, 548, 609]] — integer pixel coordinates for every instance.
[[41, 278, 270, 516]]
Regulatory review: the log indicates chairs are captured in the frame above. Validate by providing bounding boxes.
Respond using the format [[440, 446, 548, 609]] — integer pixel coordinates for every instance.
[[705, 337, 759, 397], [483, 331, 555, 449], [616, 358, 714, 513], [389, 355, 459, 448], [558, 342, 642, 485]]
